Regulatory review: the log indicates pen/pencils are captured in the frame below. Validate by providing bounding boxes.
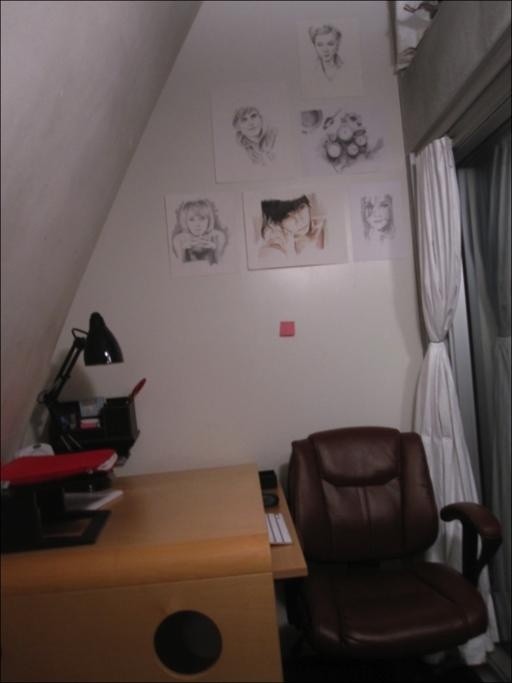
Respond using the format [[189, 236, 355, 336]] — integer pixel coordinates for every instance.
[[130, 378, 146, 399]]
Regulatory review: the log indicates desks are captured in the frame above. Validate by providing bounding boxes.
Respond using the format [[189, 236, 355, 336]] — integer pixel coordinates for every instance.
[[0, 462, 309, 681]]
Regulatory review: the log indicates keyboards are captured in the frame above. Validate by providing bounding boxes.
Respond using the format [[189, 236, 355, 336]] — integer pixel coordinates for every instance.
[[265, 511, 292, 547]]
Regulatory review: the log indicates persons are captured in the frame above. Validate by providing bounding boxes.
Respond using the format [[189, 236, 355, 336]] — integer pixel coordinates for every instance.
[[308, 24, 345, 82], [361, 193, 398, 240], [231, 104, 284, 163], [172, 197, 231, 265], [255, 193, 331, 255]]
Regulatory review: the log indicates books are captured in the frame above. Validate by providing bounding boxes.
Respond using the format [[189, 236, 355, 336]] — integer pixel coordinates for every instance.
[[63, 487, 124, 511]]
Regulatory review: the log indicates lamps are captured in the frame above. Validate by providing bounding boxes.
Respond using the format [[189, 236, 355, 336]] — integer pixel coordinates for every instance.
[[29, 308, 124, 492]]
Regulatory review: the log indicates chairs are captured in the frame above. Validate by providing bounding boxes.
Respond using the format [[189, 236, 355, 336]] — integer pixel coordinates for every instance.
[[283, 424, 503, 681]]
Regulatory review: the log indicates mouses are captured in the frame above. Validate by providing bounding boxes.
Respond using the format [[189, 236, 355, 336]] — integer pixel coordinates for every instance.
[[263, 492, 279, 508]]
